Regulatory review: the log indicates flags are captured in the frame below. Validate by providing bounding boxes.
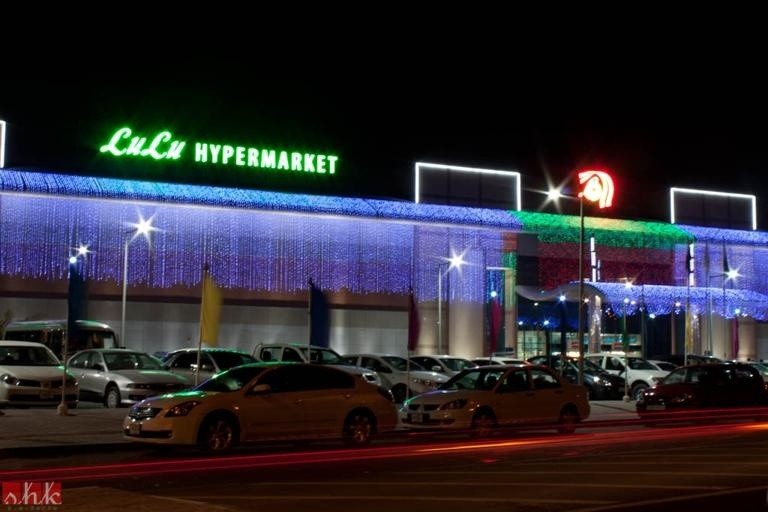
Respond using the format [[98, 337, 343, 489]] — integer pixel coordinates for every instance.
[[310, 284, 332, 349], [492, 296, 503, 352], [66, 263, 88, 350], [732, 318, 738, 358], [199, 272, 225, 347], [619, 309, 629, 353], [684, 305, 691, 353], [407, 293, 422, 351]]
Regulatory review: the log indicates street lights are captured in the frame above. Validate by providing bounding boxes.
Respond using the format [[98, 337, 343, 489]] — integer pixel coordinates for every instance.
[[710, 272, 737, 355], [56, 246, 87, 414], [579, 174, 604, 386], [120, 222, 151, 347], [438, 259, 461, 353]]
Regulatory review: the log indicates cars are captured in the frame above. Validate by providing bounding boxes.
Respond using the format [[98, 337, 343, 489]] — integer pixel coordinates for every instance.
[[0, 320, 768, 458]]
